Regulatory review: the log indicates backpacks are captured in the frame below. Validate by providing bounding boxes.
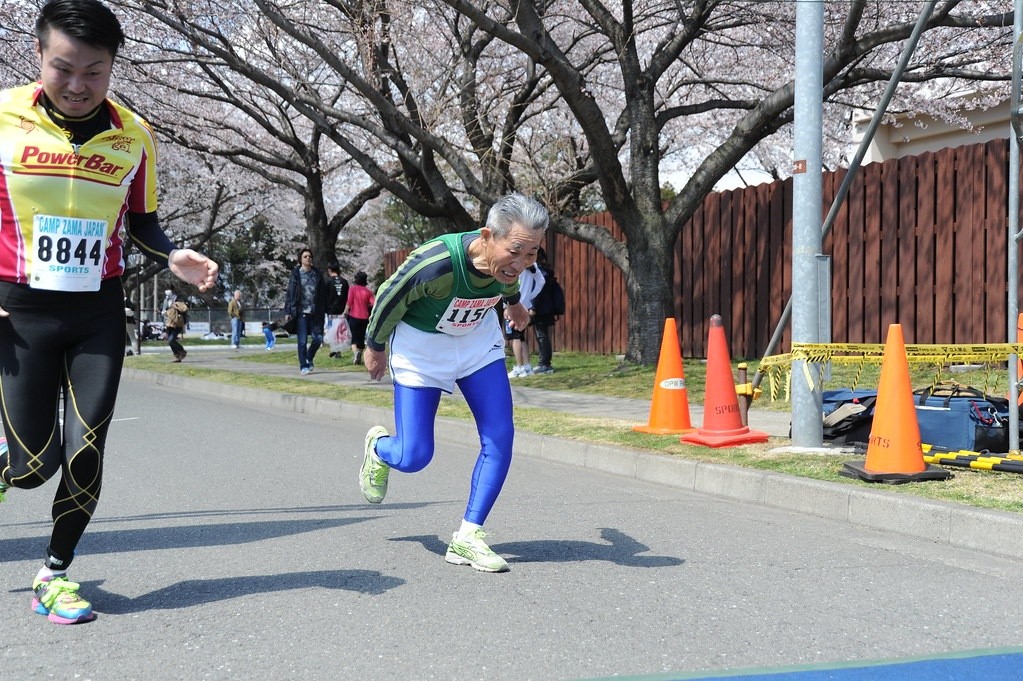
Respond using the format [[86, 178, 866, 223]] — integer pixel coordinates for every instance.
[[542, 268, 566, 314], [176, 297, 192, 329]]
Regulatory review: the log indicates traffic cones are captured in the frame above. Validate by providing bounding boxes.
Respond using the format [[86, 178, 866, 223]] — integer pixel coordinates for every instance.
[[632, 318, 696, 435], [842, 324, 950, 483], [679, 313, 770, 448]]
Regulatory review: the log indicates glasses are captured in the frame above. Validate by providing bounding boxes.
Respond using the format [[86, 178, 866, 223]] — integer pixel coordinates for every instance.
[[302, 255, 312, 259]]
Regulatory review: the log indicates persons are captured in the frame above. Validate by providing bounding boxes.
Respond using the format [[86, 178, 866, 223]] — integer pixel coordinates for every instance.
[[124, 289, 135, 356], [262, 322, 276, 351], [162, 284, 188, 363], [503, 246, 565, 379], [283, 248, 376, 376], [228, 290, 245, 350], [0, 0, 219, 625], [358, 193, 550, 574]]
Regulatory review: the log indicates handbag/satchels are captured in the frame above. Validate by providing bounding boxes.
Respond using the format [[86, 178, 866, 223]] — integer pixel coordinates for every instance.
[[330, 316, 352, 351]]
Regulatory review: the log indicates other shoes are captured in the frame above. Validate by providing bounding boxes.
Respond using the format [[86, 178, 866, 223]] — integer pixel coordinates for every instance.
[[180, 349, 187, 359], [301, 367, 312, 376], [172, 356, 181, 362], [306, 358, 314, 371], [267, 347, 272, 352], [329, 351, 342, 358], [231, 344, 244, 349]]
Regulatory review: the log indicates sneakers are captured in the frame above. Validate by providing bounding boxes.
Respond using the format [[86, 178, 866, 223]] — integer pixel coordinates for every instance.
[[30, 569, 94, 625], [445, 528, 510, 572], [353, 350, 362, 365], [533, 366, 554, 375], [359, 426, 390, 504], [0, 437, 9, 504], [507, 364, 534, 378]]
